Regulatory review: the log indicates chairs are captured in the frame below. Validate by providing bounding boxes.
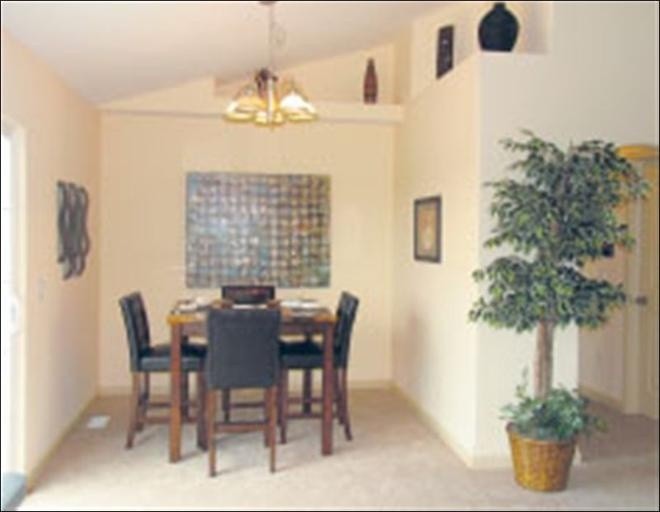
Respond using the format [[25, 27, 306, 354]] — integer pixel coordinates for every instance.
[[276, 289, 360, 448], [118, 289, 211, 455], [220, 282, 281, 419], [196, 303, 284, 480]]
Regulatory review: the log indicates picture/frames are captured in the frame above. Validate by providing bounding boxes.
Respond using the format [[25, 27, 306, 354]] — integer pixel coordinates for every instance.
[[411, 194, 443, 265]]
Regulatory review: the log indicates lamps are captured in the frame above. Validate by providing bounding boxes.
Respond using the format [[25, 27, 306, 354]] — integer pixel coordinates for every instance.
[[222, 1, 321, 131]]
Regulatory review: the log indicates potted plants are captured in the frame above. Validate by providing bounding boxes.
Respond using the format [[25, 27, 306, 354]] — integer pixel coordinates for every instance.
[[462, 128, 654, 496]]
